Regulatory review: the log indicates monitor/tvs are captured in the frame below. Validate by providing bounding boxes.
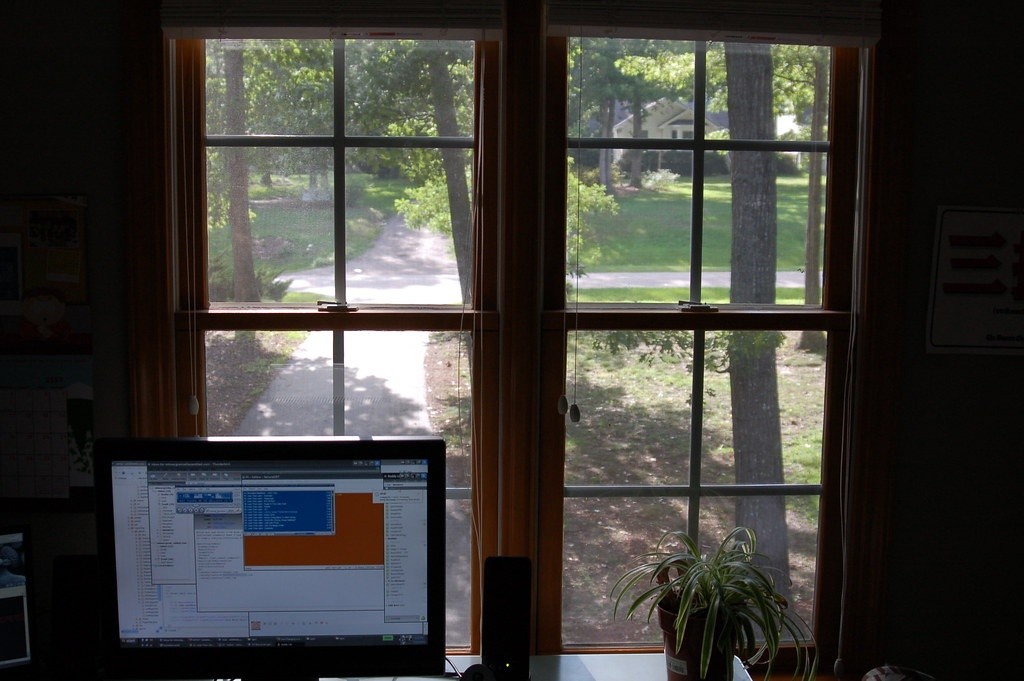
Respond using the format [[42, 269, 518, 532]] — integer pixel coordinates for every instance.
[[92, 434, 444, 681]]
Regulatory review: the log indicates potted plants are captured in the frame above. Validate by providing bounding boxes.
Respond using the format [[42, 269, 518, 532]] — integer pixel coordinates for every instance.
[[611, 526, 820, 681]]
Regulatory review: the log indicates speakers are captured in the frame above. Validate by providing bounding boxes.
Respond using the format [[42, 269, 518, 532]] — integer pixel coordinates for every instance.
[[481, 557, 531, 681]]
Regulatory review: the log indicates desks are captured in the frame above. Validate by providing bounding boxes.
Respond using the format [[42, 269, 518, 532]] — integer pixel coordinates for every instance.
[[445, 652, 754, 681]]
